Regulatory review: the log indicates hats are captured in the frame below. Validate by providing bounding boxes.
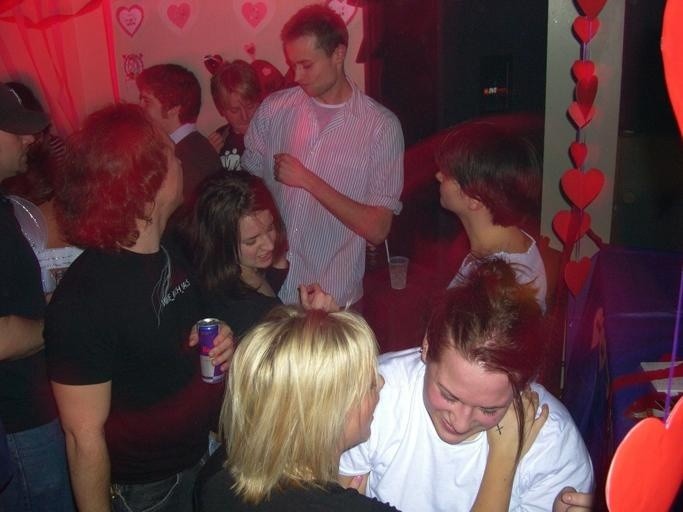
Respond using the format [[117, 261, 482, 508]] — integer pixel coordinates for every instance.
[[0, 82, 52, 134]]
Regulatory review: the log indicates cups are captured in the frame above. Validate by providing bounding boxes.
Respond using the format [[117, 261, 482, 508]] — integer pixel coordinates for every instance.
[[387, 256, 409, 290]]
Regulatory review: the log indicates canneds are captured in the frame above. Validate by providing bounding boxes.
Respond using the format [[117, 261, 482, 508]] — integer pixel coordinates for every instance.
[[196, 318, 226, 385]]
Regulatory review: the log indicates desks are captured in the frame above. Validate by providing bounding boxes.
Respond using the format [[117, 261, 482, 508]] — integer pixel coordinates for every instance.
[[560, 246, 683, 478]]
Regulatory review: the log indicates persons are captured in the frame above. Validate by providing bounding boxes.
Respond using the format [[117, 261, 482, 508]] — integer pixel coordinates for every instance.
[[0, 7, 599, 512]]
